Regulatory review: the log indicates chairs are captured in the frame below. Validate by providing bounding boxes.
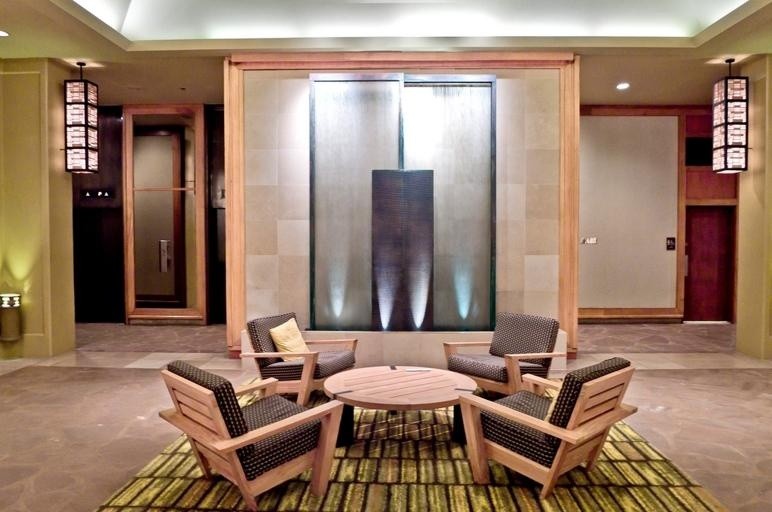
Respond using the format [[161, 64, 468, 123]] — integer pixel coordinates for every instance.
[[239, 312, 358, 406], [458, 356, 637, 500], [442, 312, 560, 396], [158, 359, 344, 512]]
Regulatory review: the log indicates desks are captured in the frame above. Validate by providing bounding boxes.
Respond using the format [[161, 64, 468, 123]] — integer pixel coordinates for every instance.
[[323, 365, 477, 447]]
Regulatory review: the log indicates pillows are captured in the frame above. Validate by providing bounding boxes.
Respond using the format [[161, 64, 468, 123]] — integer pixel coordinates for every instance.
[[270, 318, 310, 361]]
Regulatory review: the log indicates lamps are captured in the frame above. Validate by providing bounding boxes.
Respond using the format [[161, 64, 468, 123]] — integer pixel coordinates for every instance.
[[0, 294, 22, 342], [63, 62, 99, 174], [711, 58, 750, 174]]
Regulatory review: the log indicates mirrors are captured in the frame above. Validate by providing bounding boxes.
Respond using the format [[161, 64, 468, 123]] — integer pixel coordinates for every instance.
[[120, 102, 209, 327]]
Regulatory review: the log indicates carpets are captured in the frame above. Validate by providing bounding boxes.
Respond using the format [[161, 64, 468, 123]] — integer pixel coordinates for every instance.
[[89, 376, 726, 512]]
